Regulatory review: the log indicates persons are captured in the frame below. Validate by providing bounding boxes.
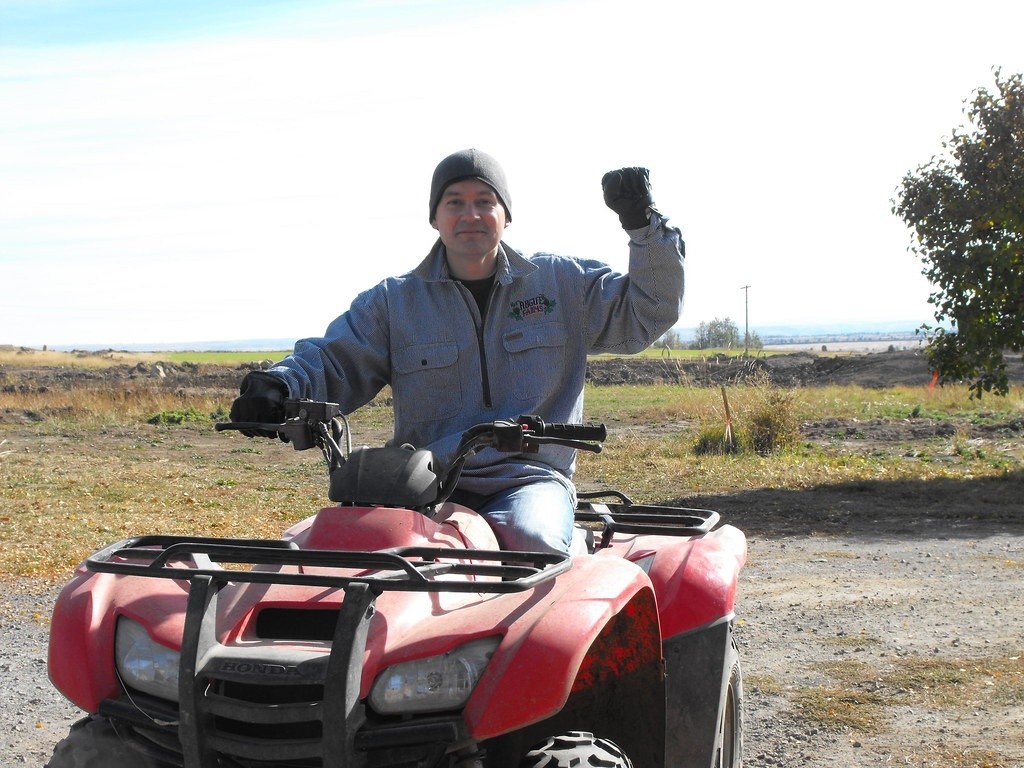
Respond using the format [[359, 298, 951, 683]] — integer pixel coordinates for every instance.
[[229, 148, 685, 581]]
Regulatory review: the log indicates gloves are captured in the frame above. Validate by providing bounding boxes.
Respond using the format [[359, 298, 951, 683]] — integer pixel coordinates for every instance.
[[600, 166, 660, 229], [224, 373, 291, 443]]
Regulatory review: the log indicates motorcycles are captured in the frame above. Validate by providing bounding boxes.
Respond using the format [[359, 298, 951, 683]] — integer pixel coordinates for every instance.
[[49, 394, 748, 768]]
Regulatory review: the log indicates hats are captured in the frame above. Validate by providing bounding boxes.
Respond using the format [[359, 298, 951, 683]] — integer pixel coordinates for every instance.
[[427, 147, 513, 223]]
